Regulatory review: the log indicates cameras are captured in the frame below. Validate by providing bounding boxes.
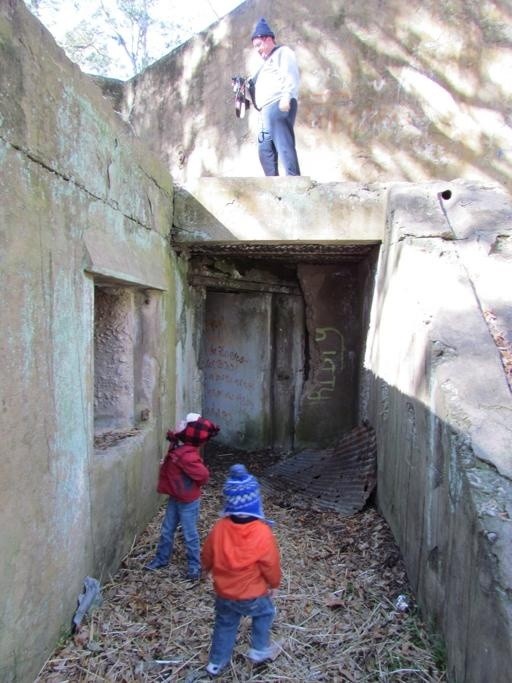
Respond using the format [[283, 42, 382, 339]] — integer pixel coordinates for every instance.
[[232, 76, 245, 92]]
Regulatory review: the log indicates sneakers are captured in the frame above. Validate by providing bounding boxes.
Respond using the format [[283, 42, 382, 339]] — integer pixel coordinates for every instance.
[[207, 659, 222, 677], [146, 559, 168, 571], [246, 648, 273, 663], [186, 567, 201, 580]]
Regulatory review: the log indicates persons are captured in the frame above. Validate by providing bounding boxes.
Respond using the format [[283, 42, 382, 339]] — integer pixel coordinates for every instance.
[[200, 462, 281, 676], [230, 15, 301, 175], [140, 412, 220, 579]]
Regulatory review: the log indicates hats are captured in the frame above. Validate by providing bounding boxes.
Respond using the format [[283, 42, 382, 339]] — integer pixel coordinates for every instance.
[[251, 18, 275, 39], [167, 413, 221, 443], [223, 464, 264, 518]]
